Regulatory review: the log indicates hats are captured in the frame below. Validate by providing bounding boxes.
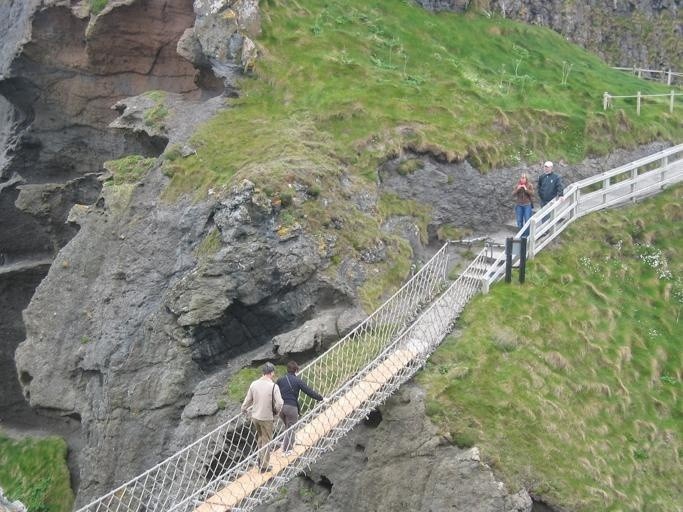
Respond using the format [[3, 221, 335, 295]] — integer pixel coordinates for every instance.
[[545, 161, 553, 168]]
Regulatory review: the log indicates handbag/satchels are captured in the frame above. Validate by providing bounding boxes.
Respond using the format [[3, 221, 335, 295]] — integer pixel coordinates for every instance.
[[273, 399, 278, 416], [297, 400, 302, 416]]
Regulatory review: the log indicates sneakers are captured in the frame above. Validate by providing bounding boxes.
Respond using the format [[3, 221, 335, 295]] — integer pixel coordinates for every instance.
[[258, 458, 272, 472], [283, 449, 292, 457]]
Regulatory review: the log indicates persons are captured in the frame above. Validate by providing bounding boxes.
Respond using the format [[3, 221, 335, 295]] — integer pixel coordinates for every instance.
[[275, 360, 329, 457], [241, 361, 284, 473], [512, 173, 534, 239], [538, 161, 563, 234]]
